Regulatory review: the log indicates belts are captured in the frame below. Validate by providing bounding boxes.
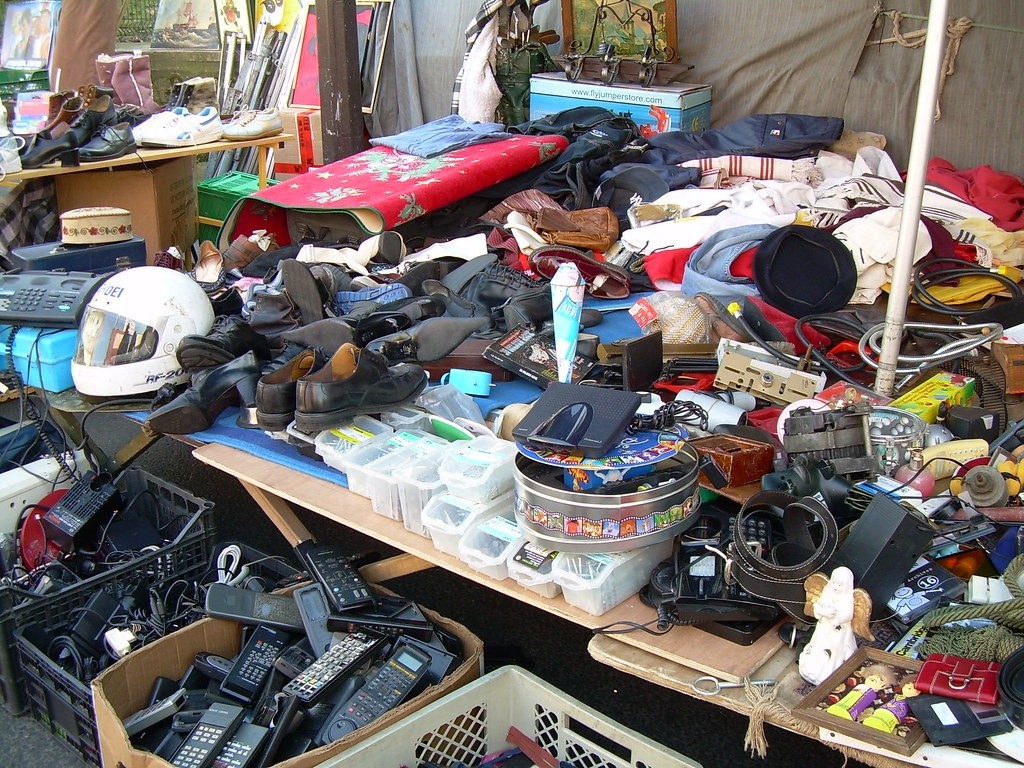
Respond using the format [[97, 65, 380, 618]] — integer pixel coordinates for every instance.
[[997, 646, 1024, 733], [704, 490, 839, 604]]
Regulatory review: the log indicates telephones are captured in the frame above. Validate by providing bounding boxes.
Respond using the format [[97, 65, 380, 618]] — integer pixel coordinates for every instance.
[[668, 509, 783, 626], [0, 267, 118, 330]]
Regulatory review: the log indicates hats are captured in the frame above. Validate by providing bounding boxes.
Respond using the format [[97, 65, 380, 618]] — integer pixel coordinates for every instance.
[[752, 224, 858, 321], [592, 166, 669, 225]]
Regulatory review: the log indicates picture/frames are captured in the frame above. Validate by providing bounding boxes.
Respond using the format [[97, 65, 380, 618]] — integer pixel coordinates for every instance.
[[789, 646, 934, 758]]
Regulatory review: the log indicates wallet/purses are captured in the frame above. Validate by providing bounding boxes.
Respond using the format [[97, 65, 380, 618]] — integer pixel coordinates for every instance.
[[914, 652, 1003, 706], [905, 695, 1013, 747]]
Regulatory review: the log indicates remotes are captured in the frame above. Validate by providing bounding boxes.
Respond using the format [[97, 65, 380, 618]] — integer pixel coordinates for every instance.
[[121, 539, 451, 767]]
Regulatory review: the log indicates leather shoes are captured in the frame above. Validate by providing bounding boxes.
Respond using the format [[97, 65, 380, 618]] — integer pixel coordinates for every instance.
[[146, 230, 629, 433]]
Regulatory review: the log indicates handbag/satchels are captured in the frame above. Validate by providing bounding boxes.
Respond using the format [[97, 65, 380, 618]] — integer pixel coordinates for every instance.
[[496, 3, 567, 124], [502, 206, 619, 251]]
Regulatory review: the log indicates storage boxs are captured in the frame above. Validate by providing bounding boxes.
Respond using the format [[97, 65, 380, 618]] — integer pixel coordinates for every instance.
[[9, 236, 147, 278], [0, 323, 81, 393], [195, 168, 285, 249], [272, 103, 324, 185], [530, 70, 713, 143], [53, 155, 199, 268], [1, 469, 701, 768]]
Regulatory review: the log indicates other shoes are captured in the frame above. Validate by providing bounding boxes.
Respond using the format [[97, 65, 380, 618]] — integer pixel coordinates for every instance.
[[0, 86, 285, 181]]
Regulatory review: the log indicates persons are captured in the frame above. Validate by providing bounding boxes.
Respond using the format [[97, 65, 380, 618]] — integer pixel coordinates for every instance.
[[863, 675, 922, 734], [827, 664, 895, 721], [803, 567, 856, 677]]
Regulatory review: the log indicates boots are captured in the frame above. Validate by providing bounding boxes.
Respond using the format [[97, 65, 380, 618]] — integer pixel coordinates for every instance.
[[95, 51, 219, 109]]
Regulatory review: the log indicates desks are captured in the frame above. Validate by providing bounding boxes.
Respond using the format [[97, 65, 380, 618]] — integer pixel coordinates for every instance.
[[31, 378, 1024, 767], [0, 132, 294, 274]]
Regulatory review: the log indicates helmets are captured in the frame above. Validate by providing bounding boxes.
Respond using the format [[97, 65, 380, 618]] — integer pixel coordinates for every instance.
[[71, 265, 215, 402]]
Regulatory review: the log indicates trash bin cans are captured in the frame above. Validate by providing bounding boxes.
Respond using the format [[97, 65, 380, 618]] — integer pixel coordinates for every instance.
[[196, 171, 282, 249]]
